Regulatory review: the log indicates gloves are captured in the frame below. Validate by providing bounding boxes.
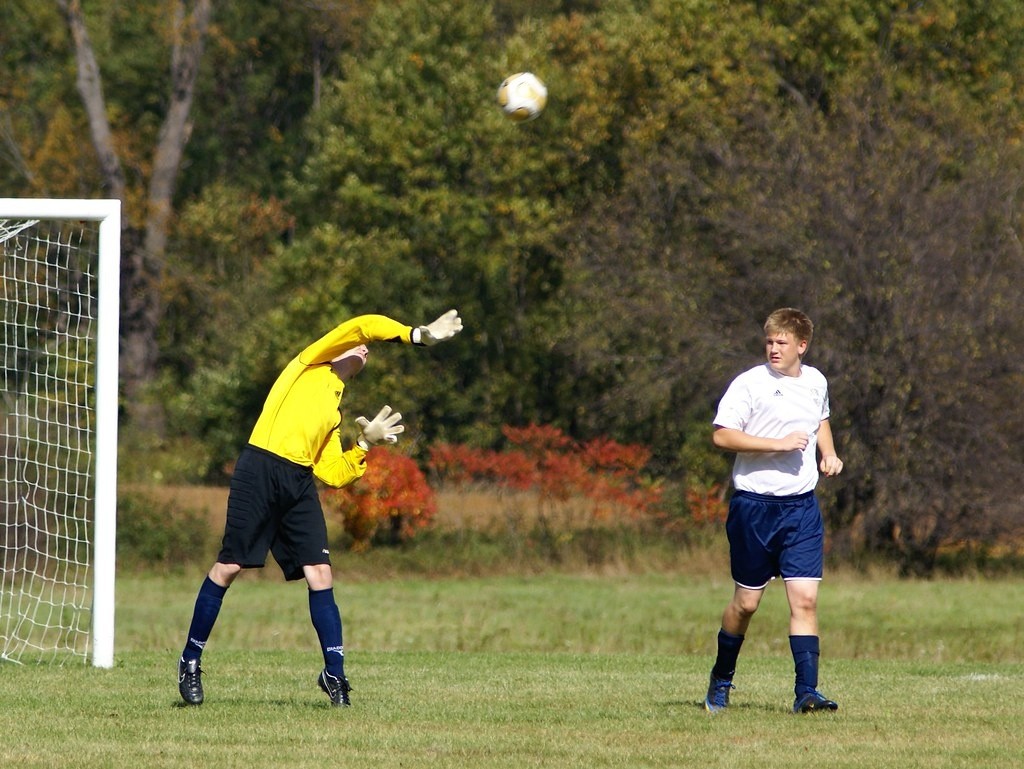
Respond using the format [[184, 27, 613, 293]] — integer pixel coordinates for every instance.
[[411, 308, 464, 347], [354, 405, 405, 451]]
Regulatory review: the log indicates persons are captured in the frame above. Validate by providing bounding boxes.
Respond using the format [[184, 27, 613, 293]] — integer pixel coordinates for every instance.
[[178, 309, 464, 709], [704, 307, 843, 715]]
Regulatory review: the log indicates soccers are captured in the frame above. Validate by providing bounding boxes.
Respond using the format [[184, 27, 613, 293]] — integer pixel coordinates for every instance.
[[495, 72, 548, 122]]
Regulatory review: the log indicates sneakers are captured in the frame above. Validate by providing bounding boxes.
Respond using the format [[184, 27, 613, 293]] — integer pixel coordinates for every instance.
[[177, 656, 207, 704], [318, 669, 354, 709], [794, 692, 837, 714], [704, 672, 736, 715]]
